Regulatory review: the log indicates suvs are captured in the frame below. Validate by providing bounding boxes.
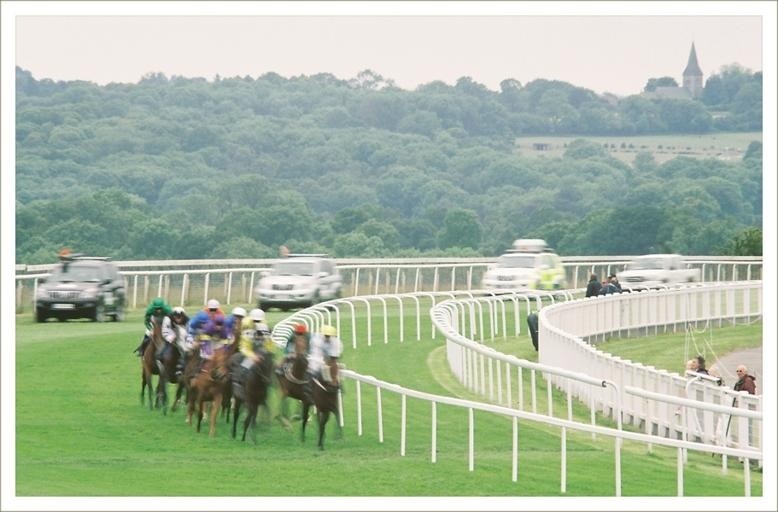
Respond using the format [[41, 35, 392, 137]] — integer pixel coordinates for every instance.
[[32, 252, 126, 324], [479, 236, 567, 301], [254, 250, 343, 311]]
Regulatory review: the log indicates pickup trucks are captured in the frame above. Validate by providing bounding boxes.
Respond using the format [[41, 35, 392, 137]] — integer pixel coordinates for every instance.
[[613, 251, 704, 294]]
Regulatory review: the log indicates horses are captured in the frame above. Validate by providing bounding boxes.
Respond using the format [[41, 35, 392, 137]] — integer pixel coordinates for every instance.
[[140, 313, 342, 449]]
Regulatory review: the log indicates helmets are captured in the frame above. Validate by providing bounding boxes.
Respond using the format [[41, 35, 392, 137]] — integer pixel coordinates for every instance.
[[249, 308, 266, 321], [294, 323, 307, 334], [321, 324, 337, 337], [172, 306, 186, 317], [152, 296, 164, 308], [207, 298, 220, 310], [232, 306, 247, 317], [254, 322, 270, 333]]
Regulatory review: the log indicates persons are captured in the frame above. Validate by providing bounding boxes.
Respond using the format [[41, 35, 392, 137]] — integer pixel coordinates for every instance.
[[130, 296, 345, 405], [732, 365, 757, 447], [690, 356, 710, 376], [580, 272, 622, 297]]
